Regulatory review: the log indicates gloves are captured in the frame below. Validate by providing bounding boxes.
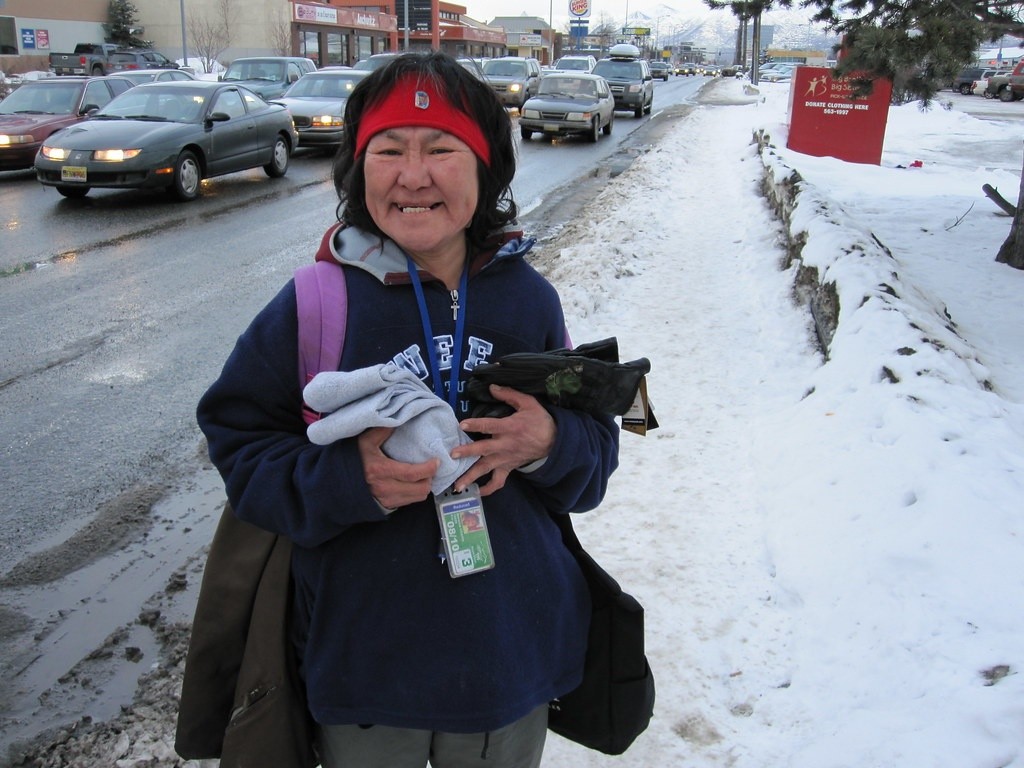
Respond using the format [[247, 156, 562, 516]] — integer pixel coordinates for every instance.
[[466, 337, 650, 441]]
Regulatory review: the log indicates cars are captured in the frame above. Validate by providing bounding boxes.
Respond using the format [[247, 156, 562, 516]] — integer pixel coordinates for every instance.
[[519, 75, 615, 142], [667, 63, 744, 77], [461, 58, 488, 76], [275, 66, 377, 149], [106, 49, 174, 75], [951, 66, 1011, 96], [759, 63, 804, 81], [109, 71, 200, 108], [0, 78, 133, 170], [33, 84, 299, 201]]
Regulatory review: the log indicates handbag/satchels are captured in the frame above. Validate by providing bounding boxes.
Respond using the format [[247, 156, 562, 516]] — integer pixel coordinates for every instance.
[[548, 513, 654, 756]]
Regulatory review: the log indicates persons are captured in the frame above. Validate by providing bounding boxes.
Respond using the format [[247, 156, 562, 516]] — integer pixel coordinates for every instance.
[[193, 49, 622, 768]]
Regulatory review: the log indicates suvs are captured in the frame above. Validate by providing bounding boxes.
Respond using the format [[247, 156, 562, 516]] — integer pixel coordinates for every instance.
[[649, 62, 669, 82], [218, 58, 317, 108], [478, 57, 543, 107], [584, 45, 654, 119], [554, 54, 596, 88], [1007, 58, 1024, 100]]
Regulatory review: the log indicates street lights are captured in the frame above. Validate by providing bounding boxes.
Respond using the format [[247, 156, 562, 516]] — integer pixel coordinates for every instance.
[[655, 15, 671, 62]]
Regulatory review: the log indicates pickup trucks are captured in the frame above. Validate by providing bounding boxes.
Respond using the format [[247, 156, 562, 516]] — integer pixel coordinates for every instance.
[[48, 44, 124, 78], [988, 74, 1023, 103]]
